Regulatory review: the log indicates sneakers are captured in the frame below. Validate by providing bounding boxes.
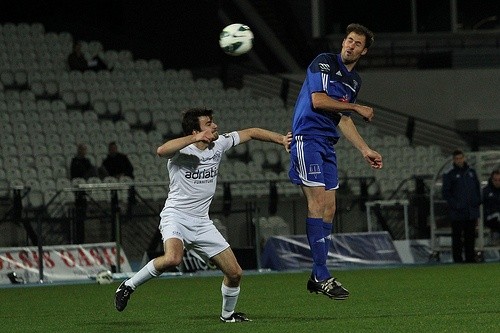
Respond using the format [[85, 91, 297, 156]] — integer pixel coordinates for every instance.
[[114, 277, 135, 312], [218, 312, 252, 323], [306, 273, 350, 300]]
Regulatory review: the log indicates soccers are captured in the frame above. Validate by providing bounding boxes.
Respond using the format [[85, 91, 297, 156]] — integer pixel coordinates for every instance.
[[219, 21, 255, 58]]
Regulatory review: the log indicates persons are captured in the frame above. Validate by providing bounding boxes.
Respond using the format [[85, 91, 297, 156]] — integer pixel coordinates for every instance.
[[69, 144, 92, 244], [442, 152, 482, 265], [480, 169, 500, 250], [289, 23, 384, 299], [102, 143, 134, 226], [114, 107, 292, 324]]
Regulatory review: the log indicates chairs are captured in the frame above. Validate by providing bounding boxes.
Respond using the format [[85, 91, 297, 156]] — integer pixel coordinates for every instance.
[[0, 24, 458, 255]]
